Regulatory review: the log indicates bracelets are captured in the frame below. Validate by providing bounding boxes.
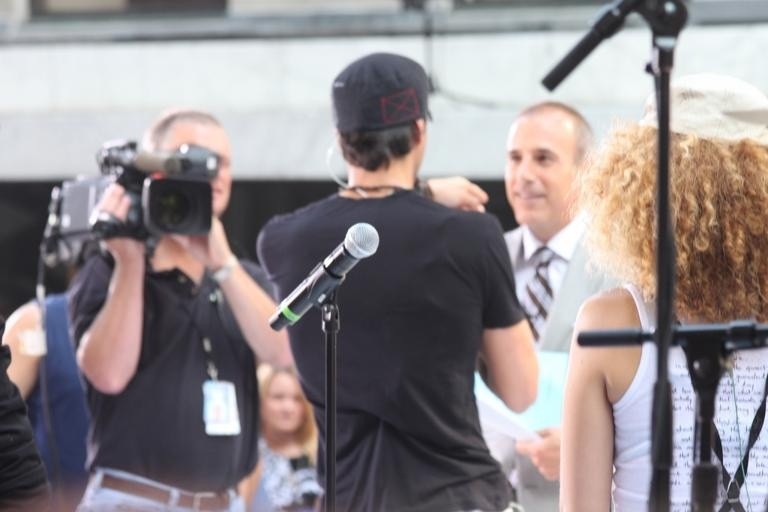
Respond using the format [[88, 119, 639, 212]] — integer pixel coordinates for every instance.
[[418, 177, 434, 200], [210, 255, 237, 282]]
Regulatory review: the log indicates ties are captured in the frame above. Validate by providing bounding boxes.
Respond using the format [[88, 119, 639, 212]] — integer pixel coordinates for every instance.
[[522, 246, 557, 343]]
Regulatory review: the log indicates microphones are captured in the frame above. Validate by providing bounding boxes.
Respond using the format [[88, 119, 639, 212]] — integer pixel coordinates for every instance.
[[268, 221, 381, 332]]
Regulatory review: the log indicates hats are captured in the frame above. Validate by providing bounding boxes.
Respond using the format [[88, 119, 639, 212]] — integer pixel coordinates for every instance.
[[329, 51, 435, 133]]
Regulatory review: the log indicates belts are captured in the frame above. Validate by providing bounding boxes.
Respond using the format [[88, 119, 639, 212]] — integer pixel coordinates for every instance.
[[99, 471, 237, 511]]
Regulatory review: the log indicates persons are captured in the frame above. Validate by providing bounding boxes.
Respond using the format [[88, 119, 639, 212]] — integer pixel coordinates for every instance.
[[557, 89, 768, 512], [3, 291, 91, 512], [472, 96, 626, 512], [1, 314, 52, 512], [254, 358, 319, 511], [64, 107, 291, 512], [254, 51, 543, 512]]
